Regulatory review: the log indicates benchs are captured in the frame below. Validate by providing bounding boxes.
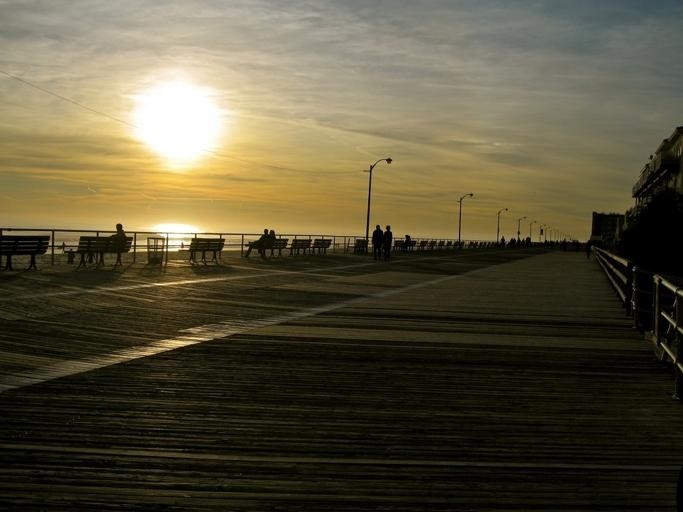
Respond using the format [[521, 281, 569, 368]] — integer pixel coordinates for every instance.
[[0, 235, 50, 272], [179, 238, 225, 266], [253, 239, 495, 258], [64, 236, 133, 272]]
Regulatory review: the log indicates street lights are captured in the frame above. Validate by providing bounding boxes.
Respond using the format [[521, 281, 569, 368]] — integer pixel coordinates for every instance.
[[457, 193, 474, 248], [529, 221, 566, 242], [496, 208, 508, 244], [365, 158, 393, 254], [518, 216, 527, 241]]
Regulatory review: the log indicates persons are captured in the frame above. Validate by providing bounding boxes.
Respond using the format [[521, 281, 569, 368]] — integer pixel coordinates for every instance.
[[372, 225, 383, 261], [257, 229, 276, 255], [111, 224, 127, 249], [405, 234, 411, 240], [500, 236, 531, 248], [382, 225, 392, 262]]
